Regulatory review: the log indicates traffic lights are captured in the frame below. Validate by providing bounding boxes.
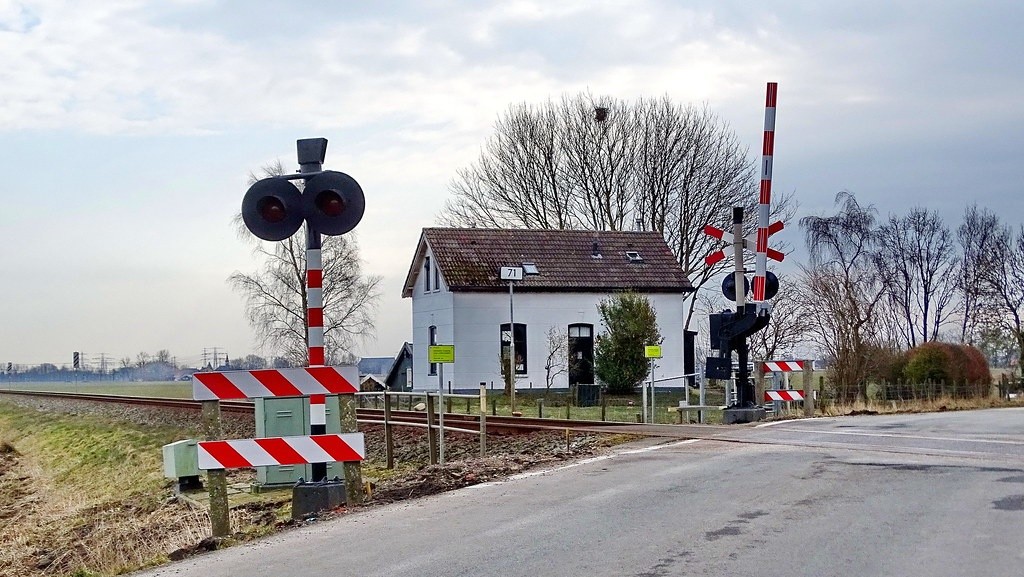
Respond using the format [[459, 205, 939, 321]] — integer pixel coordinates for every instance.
[[242, 176, 306, 241], [302, 171, 365, 236]]
